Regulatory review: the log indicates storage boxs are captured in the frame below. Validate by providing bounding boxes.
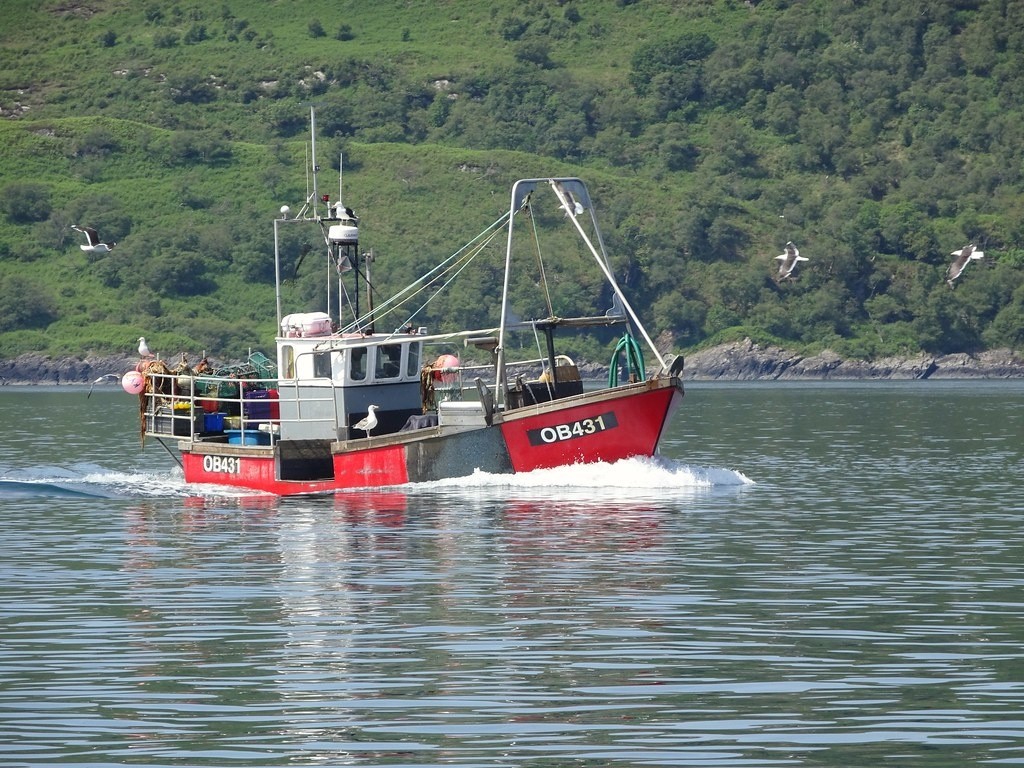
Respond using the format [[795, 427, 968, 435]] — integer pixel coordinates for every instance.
[[157, 405, 271, 447]]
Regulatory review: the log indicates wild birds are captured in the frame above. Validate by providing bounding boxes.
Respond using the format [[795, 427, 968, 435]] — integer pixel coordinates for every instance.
[[350, 404, 379, 439], [947, 243, 984, 281], [72, 225, 117, 252], [87, 373, 121, 400], [136, 337, 155, 360], [774, 241, 809, 279]]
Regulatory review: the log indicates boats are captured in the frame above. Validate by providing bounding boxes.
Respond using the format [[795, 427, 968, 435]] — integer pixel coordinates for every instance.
[[122, 107, 688, 496]]
